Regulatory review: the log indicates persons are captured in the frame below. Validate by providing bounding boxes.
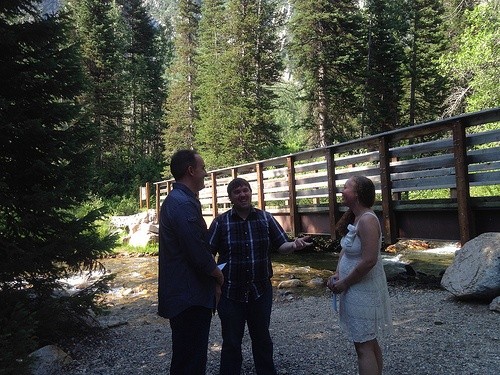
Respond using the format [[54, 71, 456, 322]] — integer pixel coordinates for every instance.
[[155, 149, 226, 375], [325, 174, 394, 375], [209, 178, 317, 375]]
